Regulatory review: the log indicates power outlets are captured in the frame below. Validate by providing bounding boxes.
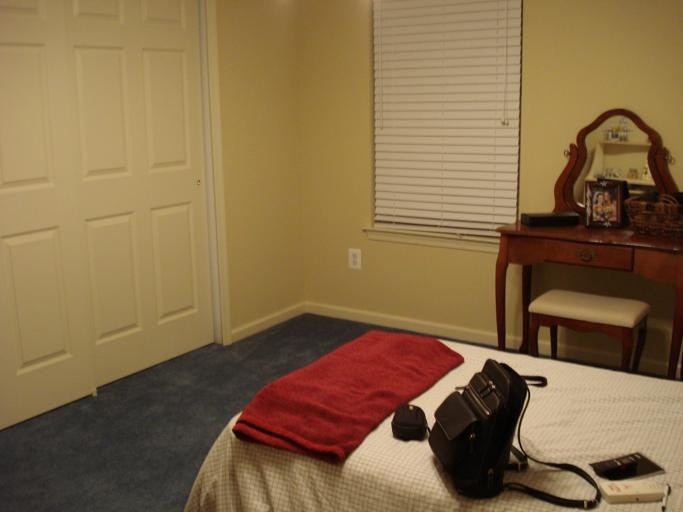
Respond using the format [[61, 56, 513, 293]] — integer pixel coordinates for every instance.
[[349, 248, 362, 270]]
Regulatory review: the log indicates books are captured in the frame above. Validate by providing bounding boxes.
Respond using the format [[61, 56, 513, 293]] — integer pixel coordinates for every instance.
[[598, 478, 665, 504]]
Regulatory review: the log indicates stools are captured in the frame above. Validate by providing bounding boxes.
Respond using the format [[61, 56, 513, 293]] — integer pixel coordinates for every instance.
[[528, 288, 652, 372]]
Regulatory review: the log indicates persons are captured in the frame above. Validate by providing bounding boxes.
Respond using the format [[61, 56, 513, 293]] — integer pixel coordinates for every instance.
[[591, 188, 617, 222]]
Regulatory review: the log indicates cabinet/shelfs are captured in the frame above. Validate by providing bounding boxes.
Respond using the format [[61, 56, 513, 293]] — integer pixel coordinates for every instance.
[[586, 139, 656, 187]]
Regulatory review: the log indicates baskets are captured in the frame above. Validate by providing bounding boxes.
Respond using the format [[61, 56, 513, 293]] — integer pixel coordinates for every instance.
[[626, 200, 683, 241]]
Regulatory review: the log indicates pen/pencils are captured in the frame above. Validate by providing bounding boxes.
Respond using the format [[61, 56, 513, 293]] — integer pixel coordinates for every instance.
[[662, 483, 671, 511]]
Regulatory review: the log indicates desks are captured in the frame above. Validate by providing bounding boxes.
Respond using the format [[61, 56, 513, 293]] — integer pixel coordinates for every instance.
[[494, 217, 683, 382]]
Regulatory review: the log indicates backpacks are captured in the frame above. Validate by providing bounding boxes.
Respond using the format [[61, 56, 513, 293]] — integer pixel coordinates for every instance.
[[429, 359, 601, 509]]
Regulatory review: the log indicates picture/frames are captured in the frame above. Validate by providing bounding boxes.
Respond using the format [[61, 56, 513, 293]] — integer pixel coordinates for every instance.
[[584, 180, 624, 228]]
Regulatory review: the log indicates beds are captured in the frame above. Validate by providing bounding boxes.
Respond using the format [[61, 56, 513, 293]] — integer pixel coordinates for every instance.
[[184, 330, 682, 512]]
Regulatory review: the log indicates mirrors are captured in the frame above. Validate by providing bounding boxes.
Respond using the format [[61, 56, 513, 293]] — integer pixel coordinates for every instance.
[[550, 108, 679, 214]]
